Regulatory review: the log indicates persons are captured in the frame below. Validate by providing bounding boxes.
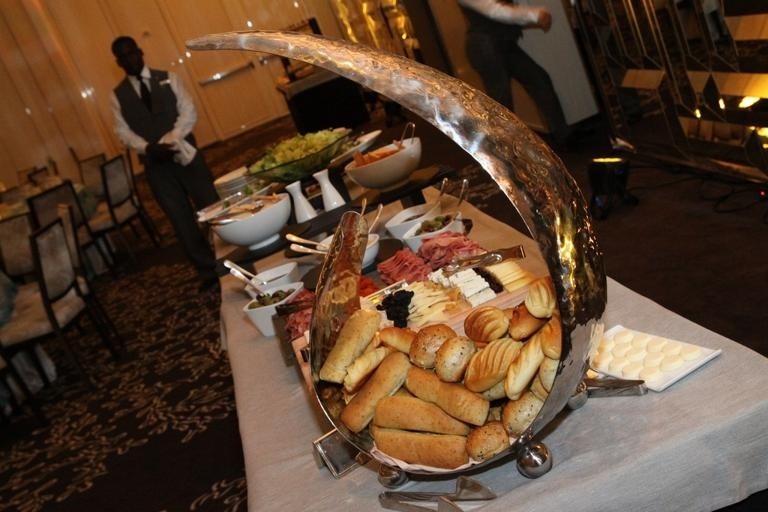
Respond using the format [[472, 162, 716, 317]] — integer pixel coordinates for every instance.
[[457, 0, 581, 166], [110, 36, 220, 289]]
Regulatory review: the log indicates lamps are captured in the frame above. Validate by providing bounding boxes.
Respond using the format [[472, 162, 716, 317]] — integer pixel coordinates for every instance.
[[586, 156, 642, 222]]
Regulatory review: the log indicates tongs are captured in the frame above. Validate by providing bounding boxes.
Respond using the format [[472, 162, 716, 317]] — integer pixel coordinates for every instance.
[[442, 244, 526, 278], [378, 474, 497, 512], [582, 378, 648, 397]]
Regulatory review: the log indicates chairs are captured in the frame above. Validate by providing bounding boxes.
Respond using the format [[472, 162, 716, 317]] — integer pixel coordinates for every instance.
[[2, 148, 165, 281], [3, 204, 127, 416]]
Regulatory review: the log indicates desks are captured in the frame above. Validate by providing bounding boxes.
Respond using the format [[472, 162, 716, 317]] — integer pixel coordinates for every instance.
[[274, 69, 371, 138]]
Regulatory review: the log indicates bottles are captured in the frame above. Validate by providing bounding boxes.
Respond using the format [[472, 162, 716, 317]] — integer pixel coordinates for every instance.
[[313, 168, 345, 213], [284, 181, 318, 225]]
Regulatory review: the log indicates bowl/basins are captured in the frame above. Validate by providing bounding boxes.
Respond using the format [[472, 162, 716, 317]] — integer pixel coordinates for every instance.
[[318, 233, 380, 272], [384, 201, 442, 242], [210, 194, 292, 252], [246, 262, 300, 298], [344, 137, 422, 193], [244, 282, 304, 336], [243, 128, 352, 184], [403, 212, 466, 254]]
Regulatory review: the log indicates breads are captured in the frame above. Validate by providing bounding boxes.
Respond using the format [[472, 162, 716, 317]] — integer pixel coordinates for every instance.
[[593, 331, 700, 381], [319, 276, 563, 470]]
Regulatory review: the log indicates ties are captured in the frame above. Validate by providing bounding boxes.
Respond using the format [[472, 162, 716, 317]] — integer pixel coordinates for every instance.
[[137, 75, 151, 111]]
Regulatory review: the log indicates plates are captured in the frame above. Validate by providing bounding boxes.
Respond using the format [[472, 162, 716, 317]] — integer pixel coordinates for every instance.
[[194, 182, 282, 222], [212, 164, 270, 202], [329, 130, 381, 167]]
